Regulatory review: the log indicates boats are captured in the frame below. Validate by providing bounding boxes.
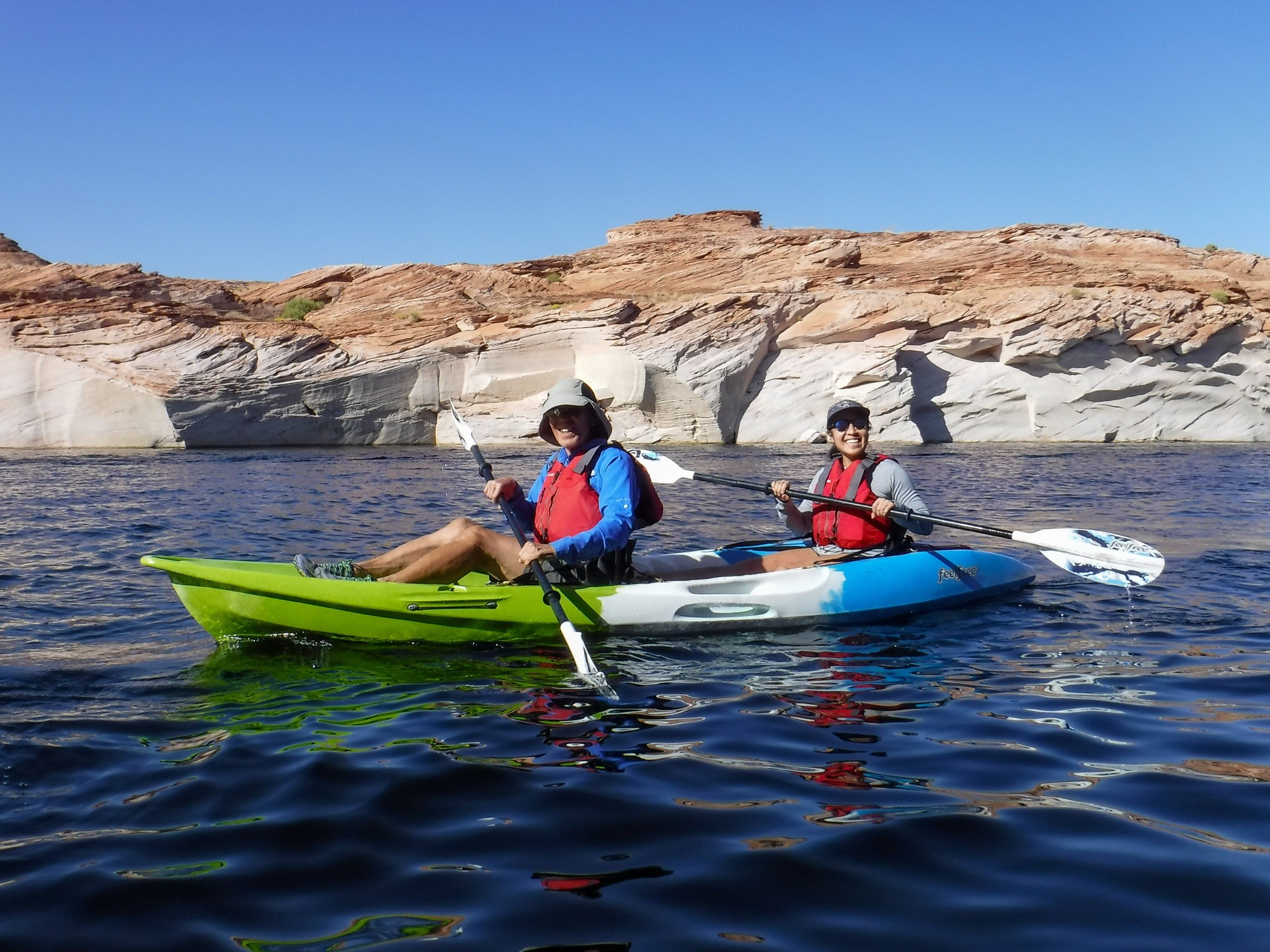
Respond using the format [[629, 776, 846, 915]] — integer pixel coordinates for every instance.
[[140, 537, 1039, 658]]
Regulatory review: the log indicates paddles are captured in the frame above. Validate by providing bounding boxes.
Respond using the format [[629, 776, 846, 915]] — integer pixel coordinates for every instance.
[[625, 445, 1167, 591], [447, 393, 599, 677]]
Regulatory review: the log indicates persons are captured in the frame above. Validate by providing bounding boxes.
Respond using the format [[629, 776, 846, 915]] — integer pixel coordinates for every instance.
[[620, 400, 932, 586], [288, 378, 642, 585]]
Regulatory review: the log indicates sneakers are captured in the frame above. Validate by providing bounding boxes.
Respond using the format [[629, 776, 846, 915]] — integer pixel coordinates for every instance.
[[293, 554, 356, 578], [313, 568, 378, 583]]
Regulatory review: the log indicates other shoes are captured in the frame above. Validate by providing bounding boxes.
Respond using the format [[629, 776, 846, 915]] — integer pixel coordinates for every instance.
[[623, 567, 663, 585]]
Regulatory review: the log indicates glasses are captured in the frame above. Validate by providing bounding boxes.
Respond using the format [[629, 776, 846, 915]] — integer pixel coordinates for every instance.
[[544, 405, 590, 419], [831, 418, 870, 433]]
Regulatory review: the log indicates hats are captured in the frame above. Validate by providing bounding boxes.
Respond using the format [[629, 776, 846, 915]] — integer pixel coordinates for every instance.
[[539, 379, 614, 440], [827, 400, 870, 424]]
